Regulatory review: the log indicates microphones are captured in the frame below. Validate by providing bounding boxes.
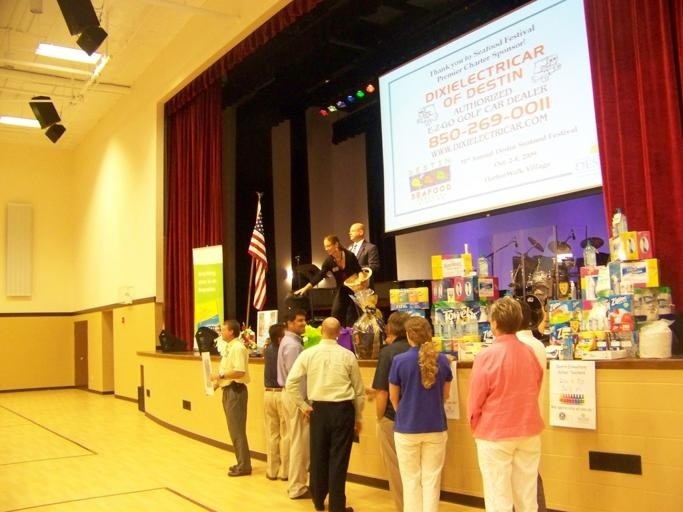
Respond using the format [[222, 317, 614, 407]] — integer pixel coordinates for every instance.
[[295, 255, 301, 262], [571, 229, 576, 240], [513, 235, 519, 248]]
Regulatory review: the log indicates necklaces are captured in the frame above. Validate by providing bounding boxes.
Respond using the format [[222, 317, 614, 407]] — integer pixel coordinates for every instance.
[[336, 252, 342, 264]]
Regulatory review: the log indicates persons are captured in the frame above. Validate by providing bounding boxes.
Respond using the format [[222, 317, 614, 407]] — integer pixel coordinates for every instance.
[[285, 316, 365, 511], [264, 308, 311, 499], [211, 319, 251, 476], [343, 223, 380, 292], [467, 296, 547, 511], [365, 311, 453, 511], [293, 234, 364, 328]]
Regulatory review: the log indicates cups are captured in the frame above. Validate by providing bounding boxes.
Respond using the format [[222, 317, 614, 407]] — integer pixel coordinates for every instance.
[[579, 316, 607, 331]]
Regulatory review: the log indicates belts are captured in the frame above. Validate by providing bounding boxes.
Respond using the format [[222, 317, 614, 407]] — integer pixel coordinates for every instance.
[[265, 388, 283, 392]]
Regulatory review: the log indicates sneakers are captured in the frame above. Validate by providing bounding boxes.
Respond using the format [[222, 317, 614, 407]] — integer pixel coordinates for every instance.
[[266, 473, 354, 512]]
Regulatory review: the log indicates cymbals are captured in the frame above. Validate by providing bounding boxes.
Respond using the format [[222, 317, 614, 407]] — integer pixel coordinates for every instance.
[[548, 241, 571, 254], [581, 237, 604, 249], [528, 237, 544, 252]]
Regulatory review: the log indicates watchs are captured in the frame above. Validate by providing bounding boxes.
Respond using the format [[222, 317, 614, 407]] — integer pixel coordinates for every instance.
[[219, 371, 224, 379]]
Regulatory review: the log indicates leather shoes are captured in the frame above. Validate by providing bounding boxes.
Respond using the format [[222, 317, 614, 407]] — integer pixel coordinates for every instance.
[[229, 469, 251, 476], [228, 465, 252, 471]]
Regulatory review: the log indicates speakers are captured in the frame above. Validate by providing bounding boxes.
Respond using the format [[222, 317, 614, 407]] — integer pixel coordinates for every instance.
[[76, 27, 108, 56], [195, 326, 220, 355], [29, 96, 64, 129], [158, 330, 183, 352], [44, 123, 66, 144], [57, 0, 100, 36]]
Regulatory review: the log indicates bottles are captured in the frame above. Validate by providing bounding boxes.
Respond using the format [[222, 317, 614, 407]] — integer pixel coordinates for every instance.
[[611, 207, 627, 237], [582, 240, 596, 267], [596, 341, 631, 350], [476, 253, 488, 276], [560, 334, 573, 360]]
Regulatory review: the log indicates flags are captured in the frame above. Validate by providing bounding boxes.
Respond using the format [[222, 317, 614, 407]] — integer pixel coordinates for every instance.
[[248, 202, 268, 310]]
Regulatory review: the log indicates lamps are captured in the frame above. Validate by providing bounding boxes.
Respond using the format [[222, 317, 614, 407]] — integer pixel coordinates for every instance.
[[57, 0, 108, 55], [29, 95, 66, 143]]
